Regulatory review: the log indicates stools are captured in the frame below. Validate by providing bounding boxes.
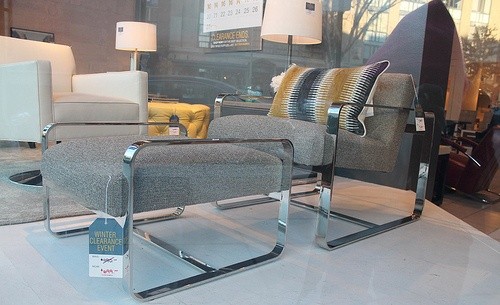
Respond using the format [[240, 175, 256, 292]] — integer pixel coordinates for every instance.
[[40, 121, 294, 302]]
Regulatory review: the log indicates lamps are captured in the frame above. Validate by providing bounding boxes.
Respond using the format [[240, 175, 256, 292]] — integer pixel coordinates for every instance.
[[259, 0, 322, 67], [115, 21, 156, 70]]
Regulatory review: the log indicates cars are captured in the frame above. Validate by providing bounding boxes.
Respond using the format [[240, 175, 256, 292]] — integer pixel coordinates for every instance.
[[145, 74, 314, 167]]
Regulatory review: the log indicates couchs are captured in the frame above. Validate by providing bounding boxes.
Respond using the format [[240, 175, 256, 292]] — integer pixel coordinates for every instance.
[[0, 35, 148, 149], [148, 102, 212, 139]]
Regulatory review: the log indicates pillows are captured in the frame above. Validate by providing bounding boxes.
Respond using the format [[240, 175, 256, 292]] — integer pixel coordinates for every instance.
[[266, 60, 390, 137]]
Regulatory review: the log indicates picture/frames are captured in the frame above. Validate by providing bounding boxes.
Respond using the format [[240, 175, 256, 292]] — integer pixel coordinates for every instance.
[[10, 27, 55, 44]]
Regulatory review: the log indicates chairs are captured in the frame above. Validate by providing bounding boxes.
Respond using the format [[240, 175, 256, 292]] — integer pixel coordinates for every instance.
[[440, 125, 500, 205], [207, 72, 435, 251]]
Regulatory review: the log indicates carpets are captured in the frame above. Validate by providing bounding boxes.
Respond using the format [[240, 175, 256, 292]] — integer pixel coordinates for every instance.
[[0, 142, 98, 226]]
